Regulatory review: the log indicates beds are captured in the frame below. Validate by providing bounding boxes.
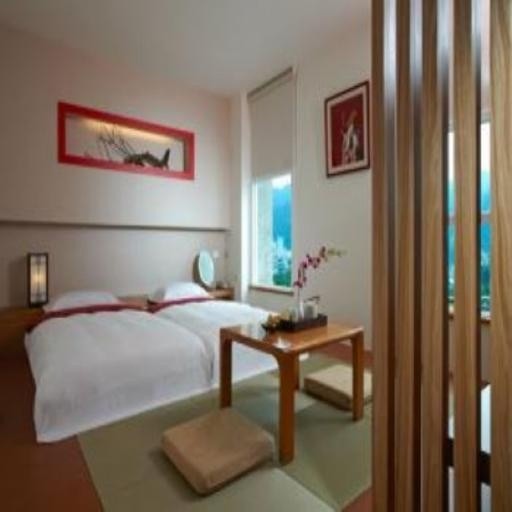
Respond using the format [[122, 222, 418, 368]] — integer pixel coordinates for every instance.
[[145, 285, 308, 387], [21, 291, 209, 444]]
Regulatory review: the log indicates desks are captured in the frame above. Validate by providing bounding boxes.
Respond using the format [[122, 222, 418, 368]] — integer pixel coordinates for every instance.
[[220, 317, 365, 464]]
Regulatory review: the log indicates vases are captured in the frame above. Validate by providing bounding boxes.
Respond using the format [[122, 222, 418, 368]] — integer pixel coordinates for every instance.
[[291, 287, 305, 322]]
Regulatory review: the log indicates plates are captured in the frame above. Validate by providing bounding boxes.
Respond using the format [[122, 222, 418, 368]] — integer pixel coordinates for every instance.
[[196, 249, 215, 286]]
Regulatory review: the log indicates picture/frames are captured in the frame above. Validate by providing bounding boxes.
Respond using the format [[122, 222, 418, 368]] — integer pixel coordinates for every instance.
[[27, 251, 48, 308], [323, 79, 373, 180]]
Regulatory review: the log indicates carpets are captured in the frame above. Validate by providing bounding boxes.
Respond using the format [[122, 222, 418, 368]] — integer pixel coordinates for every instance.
[[77, 351, 453, 511]]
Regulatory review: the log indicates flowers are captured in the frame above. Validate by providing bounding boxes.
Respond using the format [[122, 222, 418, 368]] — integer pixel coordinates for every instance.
[[293, 245, 346, 287]]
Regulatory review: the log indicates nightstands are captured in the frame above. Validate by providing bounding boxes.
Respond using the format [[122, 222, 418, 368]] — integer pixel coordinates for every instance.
[[210, 287, 236, 304]]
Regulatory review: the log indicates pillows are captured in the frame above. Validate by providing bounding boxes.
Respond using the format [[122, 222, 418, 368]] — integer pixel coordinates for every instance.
[[160, 407, 273, 496], [162, 286, 207, 303], [305, 365, 373, 411], [43, 290, 117, 317]]
